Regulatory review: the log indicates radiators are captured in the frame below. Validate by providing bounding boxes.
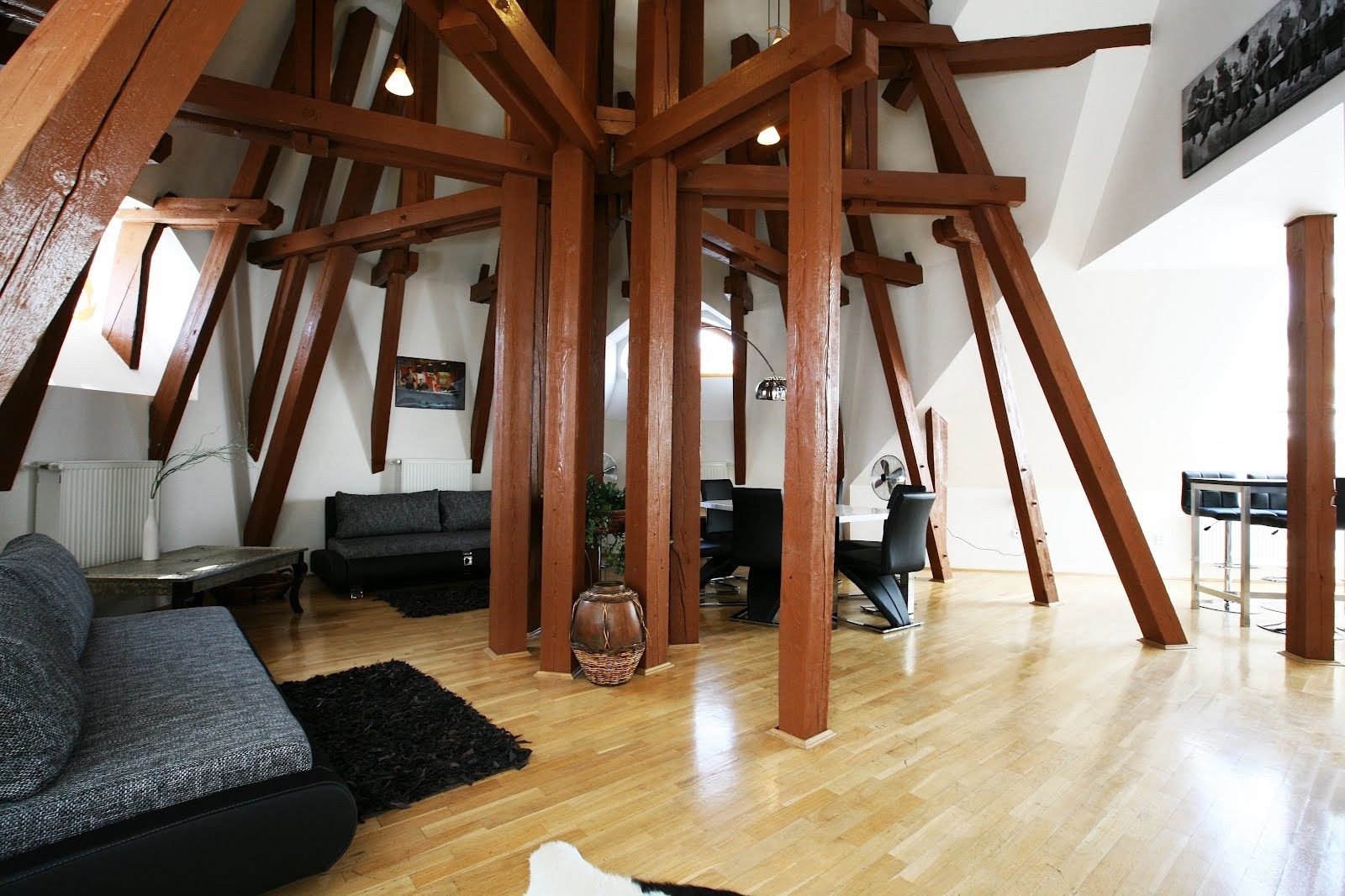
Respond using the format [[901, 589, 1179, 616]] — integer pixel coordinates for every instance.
[[35, 460, 162, 569]]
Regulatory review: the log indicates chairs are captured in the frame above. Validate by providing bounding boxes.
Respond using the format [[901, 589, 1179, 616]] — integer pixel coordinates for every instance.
[[700, 479, 937, 634]]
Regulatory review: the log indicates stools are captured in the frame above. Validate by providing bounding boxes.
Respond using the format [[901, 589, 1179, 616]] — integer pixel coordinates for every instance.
[[1248, 474, 1288, 613], [1181, 471, 1257, 614]]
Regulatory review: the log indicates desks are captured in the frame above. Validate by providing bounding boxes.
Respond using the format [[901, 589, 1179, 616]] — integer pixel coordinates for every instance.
[[78, 546, 308, 614], [1189, 479, 1287, 626], [700, 499, 890, 524]]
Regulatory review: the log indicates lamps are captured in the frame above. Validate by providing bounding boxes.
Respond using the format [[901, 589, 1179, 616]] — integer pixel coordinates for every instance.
[[385, 0, 414, 97], [766, 0, 790, 45], [701, 326, 787, 401]]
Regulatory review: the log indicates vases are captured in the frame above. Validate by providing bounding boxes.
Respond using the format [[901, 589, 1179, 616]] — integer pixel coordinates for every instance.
[[142, 498, 160, 561]]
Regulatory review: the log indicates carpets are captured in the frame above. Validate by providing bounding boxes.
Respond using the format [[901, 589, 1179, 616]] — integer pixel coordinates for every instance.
[[381, 581, 492, 620], [277, 657, 534, 824]]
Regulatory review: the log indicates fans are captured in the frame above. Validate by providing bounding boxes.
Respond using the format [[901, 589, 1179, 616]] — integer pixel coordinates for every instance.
[[603, 453, 618, 485], [872, 454, 906, 501]]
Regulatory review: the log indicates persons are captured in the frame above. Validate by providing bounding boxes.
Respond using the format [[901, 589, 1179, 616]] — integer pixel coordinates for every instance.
[[417, 379, 429, 391], [446, 381, 455, 391], [401, 367, 416, 389]]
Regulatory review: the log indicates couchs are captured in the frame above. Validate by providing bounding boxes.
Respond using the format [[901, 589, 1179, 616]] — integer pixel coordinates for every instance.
[[310, 496, 492, 597], [0, 533, 359, 896]]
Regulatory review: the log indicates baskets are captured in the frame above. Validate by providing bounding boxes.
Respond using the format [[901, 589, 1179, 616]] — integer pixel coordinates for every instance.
[[569, 640, 647, 686]]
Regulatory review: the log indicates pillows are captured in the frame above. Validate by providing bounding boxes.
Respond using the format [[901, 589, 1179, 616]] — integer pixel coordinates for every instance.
[[438, 491, 494, 533], [335, 490, 441, 537]]
